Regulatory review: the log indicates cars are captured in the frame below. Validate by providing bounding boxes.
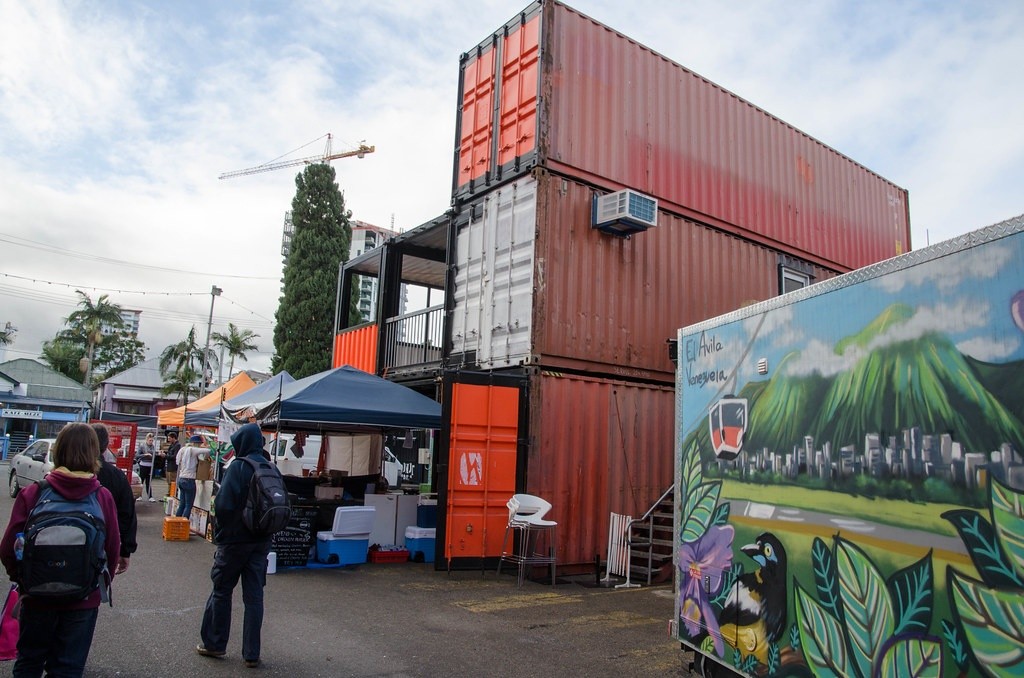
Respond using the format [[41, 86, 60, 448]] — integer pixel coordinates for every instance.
[[8, 438, 144, 503]]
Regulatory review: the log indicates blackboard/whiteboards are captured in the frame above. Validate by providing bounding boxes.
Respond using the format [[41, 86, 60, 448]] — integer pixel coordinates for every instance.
[[269, 494, 315, 566]]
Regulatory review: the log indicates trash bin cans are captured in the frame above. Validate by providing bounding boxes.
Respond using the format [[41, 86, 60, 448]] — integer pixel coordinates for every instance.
[[154, 455, 164, 477]]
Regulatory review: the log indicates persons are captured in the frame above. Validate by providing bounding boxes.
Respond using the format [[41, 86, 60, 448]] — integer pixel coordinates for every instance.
[[263, 436, 270, 462], [195, 423, 282, 668], [89, 423, 138, 575], [136, 432, 157, 502], [158, 432, 180, 502], [0, 421, 121, 678], [176, 435, 212, 536]]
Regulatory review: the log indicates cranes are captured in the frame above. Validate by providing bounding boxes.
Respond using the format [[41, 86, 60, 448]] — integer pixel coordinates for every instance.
[[217, 131, 375, 183]]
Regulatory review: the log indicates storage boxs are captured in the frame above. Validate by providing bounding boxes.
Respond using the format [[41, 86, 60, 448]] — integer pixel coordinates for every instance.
[[196, 460, 210, 480], [163, 481, 176, 515], [266, 553, 277, 574], [404, 525, 436, 564], [370, 549, 409, 564], [316, 505, 376, 565], [162, 517, 191, 542], [314, 486, 343, 500]]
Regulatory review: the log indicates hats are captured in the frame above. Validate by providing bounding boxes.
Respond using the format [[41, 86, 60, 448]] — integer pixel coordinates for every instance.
[[189, 435, 204, 443]]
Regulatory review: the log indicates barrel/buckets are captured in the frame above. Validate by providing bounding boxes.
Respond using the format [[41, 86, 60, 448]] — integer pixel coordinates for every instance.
[[197, 459, 210, 480]]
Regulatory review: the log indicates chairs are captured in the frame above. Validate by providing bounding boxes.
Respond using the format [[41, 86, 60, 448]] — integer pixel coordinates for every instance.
[[496, 494, 558, 590]]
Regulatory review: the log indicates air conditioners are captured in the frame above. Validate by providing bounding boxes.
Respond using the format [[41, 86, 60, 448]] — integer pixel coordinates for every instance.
[[591, 189, 658, 237]]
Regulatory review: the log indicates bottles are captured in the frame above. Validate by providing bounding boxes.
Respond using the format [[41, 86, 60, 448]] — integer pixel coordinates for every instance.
[[14, 532, 25, 560]]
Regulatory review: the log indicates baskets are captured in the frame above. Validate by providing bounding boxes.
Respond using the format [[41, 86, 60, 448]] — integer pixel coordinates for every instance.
[[369, 551, 409, 563]]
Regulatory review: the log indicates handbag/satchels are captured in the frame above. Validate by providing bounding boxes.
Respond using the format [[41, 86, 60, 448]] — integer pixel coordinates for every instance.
[[0, 584, 19, 660]]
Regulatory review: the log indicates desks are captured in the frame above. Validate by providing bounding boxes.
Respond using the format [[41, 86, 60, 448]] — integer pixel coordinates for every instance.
[[364, 494, 417, 547]]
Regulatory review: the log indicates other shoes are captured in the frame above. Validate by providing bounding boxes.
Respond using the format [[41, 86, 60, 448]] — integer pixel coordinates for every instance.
[[196, 644, 226, 655], [246, 658, 259, 667], [149, 497, 155, 502], [136, 496, 143, 500]]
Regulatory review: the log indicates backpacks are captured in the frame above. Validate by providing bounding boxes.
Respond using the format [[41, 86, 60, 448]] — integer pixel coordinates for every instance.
[[14, 480, 106, 602], [231, 457, 291, 536]]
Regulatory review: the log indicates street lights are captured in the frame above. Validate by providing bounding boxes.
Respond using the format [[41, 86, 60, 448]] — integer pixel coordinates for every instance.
[[199, 284, 222, 401]]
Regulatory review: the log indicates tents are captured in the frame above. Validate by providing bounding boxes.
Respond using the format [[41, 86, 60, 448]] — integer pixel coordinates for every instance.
[[145, 363, 444, 504]]
